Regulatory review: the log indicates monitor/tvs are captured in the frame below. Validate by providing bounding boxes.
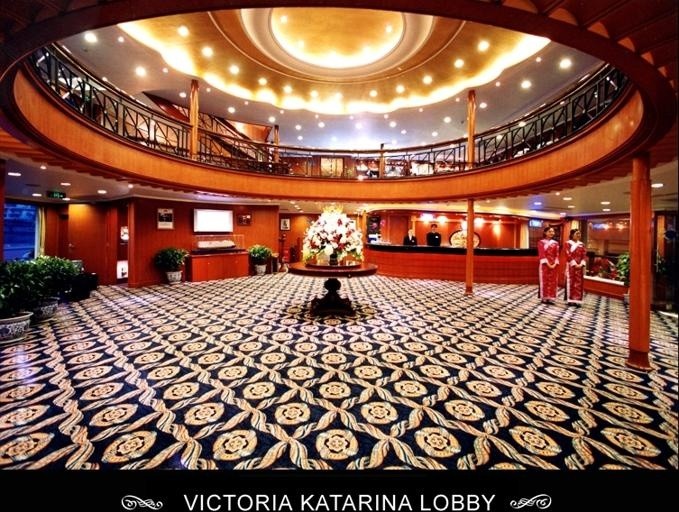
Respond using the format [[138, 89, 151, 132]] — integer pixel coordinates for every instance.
[[193, 208, 234, 233]]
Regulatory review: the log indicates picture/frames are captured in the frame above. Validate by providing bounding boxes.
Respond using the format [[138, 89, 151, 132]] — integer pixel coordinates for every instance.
[[279, 217, 291, 232], [156, 207, 175, 231]]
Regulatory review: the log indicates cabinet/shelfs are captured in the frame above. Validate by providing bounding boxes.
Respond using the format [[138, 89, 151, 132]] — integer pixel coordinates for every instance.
[[185, 252, 250, 282]]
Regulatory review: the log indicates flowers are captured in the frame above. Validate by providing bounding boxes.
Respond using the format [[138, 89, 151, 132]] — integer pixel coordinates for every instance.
[[301, 203, 365, 266]]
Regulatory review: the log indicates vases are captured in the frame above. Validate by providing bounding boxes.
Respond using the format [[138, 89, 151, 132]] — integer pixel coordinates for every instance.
[[330, 254, 338, 265]]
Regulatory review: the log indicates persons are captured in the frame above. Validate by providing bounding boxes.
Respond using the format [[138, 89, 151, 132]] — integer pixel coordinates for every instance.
[[536, 226, 562, 306], [425, 223, 442, 246], [403, 229, 419, 244], [563, 229, 587, 308]]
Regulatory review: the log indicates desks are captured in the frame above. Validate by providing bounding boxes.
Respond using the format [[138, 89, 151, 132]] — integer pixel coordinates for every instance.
[[288, 262, 378, 316]]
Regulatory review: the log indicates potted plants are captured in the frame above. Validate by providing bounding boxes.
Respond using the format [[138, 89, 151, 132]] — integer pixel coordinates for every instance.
[[616, 246, 667, 318], [154, 248, 189, 284], [0, 260, 36, 340], [34, 255, 84, 320], [250, 244, 272, 276]]
